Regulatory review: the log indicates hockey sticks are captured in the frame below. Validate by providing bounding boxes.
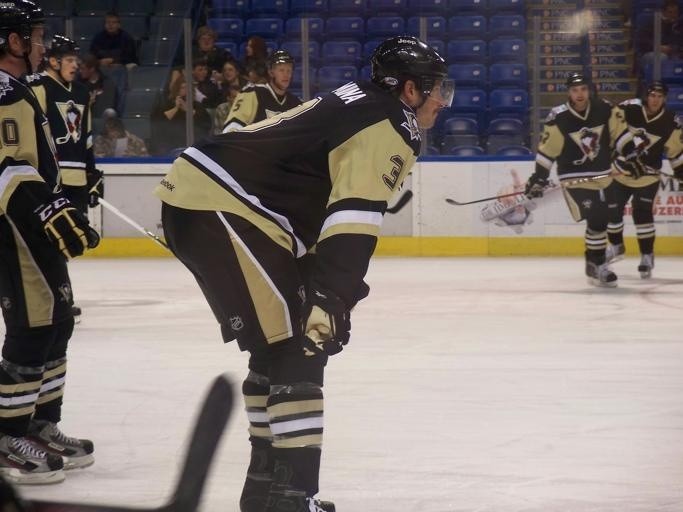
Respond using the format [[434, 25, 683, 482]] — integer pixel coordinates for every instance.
[[446, 172, 613, 205], [18, 374, 234, 510]]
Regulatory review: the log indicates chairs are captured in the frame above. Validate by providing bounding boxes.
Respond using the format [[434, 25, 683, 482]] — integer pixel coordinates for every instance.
[[622, 0, 683, 113]]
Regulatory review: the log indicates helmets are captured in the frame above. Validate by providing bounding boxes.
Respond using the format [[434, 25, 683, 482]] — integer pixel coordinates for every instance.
[[564, 73, 590, 90], [648, 80, 669, 95], [0, 0, 49, 31], [370, 34, 456, 109], [270, 49, 296, 67], [43, 34, 81, 60]]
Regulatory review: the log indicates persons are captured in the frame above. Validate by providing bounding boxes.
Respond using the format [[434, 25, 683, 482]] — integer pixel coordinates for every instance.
[[223, 48, 304, 134], [39, 12, 270, 159], [153, 34, 456, 512], [27, 33, 107, 317], [605, 83, 683, 276], [638, 2, 681, 77], [524, 73, 648, 284], [0, 1, 103, 476]]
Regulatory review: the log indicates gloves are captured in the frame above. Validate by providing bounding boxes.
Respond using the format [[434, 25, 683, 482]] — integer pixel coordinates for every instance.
[[302, 284, 351, 359], [31, 192, 101, 263], [85, 167, 106, 209]]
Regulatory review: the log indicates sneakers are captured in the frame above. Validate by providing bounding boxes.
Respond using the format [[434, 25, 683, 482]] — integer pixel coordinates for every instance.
[[637, 254, 655, 272], [0, 433, 64, 474], [24, 420, 94, 457], [262, 491, 336, 512], [584, 260, 617, 282], [240, 476, 273, 512], [604, 243, 625, 260]]
